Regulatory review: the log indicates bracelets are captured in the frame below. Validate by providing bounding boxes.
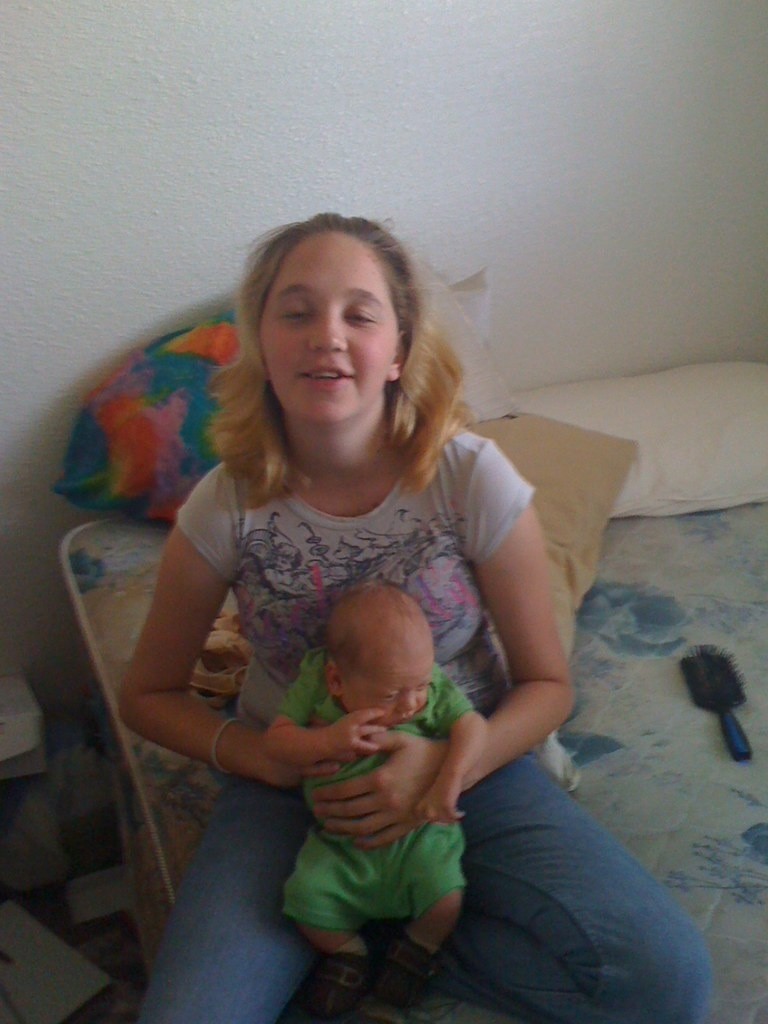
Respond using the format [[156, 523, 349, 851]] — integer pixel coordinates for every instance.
[[210, 717, 236, 778]]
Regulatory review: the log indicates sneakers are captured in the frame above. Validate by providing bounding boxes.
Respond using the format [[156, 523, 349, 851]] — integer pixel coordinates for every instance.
[[302, 947, 376, 1020], [371, 928, 444, 1007]]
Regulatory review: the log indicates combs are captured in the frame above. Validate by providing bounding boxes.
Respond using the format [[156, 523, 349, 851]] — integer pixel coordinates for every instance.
[[677, 634, 754, 766]]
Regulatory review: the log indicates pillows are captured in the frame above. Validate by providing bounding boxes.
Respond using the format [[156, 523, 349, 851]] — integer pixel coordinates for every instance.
[[494, 357, 766, 516], [456, 407, 644, 677], [53, 300, 263, 523]]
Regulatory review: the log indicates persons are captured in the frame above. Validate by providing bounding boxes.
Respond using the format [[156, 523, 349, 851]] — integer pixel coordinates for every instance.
[[108, 207, 714, 1024], [264, 583, 487, 1012]]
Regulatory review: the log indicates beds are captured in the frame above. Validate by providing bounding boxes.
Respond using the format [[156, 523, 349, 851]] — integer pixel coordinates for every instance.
[[59, 481, 766, 1021]]
